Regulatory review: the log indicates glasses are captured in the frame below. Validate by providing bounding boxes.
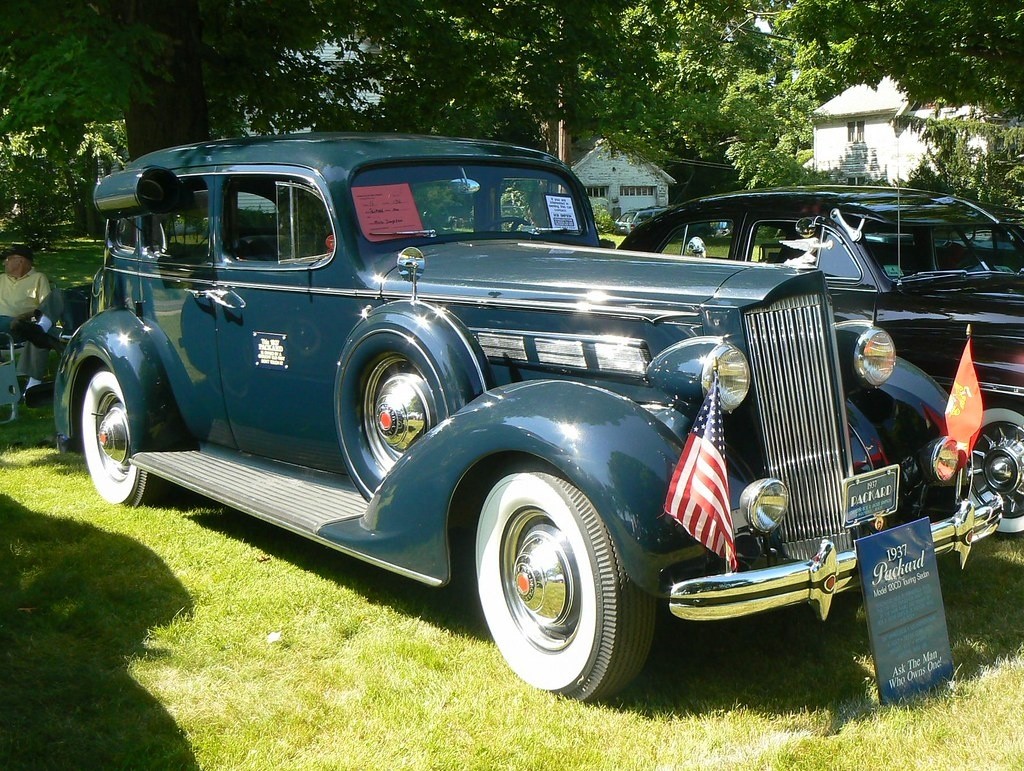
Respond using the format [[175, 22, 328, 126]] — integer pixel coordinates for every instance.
[[5, 255, 23, 261]]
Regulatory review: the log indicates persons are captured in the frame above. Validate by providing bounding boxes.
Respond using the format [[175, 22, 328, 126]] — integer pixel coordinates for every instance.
[[0, 244, 57, 403], [11, 288, 90, 403]]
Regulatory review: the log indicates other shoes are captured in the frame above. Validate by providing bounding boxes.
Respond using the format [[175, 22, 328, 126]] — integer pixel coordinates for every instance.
[[13, 320, 47, 348], [25, 382, 54, 407]]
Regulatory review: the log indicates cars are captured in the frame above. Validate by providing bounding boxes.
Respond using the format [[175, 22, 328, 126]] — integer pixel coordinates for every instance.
[[50, 130, 1006, 704], [614, 179, 1024, 542], [615, 204, 710, 237]]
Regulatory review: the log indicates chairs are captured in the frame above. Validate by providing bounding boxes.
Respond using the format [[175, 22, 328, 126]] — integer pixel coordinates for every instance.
[[239, 233, 279, 261], [0, 288, 91, 426]]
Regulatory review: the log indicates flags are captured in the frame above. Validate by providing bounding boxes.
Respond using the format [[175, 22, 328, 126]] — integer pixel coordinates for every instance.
[[945, 324, 984, 471], [663, 357, 736, 573]]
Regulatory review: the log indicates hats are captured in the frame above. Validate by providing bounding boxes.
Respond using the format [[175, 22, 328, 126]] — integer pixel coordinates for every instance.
[[0, 244, 33, 259]]
